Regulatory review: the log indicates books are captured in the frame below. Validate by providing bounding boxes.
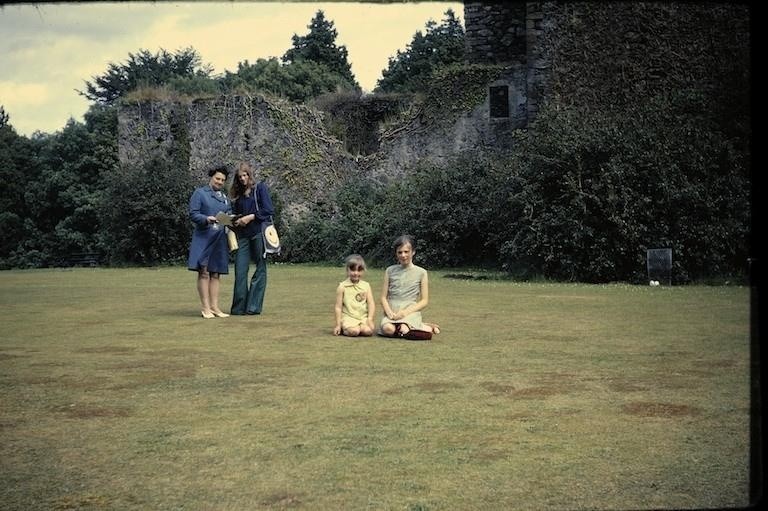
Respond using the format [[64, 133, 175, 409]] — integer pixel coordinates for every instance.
[[213, 210, 236, 226]]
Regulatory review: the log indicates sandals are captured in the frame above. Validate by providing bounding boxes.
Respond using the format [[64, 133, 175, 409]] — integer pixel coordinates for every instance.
[[423, 323, 441, 335]]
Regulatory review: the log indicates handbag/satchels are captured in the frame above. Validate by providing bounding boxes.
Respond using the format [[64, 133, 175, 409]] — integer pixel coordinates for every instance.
[[226, 228, 239, 254], [260, 222, 282, 260]]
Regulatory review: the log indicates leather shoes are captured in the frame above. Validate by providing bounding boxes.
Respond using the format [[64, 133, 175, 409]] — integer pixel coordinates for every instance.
[[210, 309, 230, 319], [201, 310, 215, 319]]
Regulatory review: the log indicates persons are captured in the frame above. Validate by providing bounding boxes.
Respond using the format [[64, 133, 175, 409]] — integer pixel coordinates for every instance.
[[187, 166, 235, 319], [332, 253, 376, 337], [377, 234, 442, 338], [225, 161, 277, 317]]
